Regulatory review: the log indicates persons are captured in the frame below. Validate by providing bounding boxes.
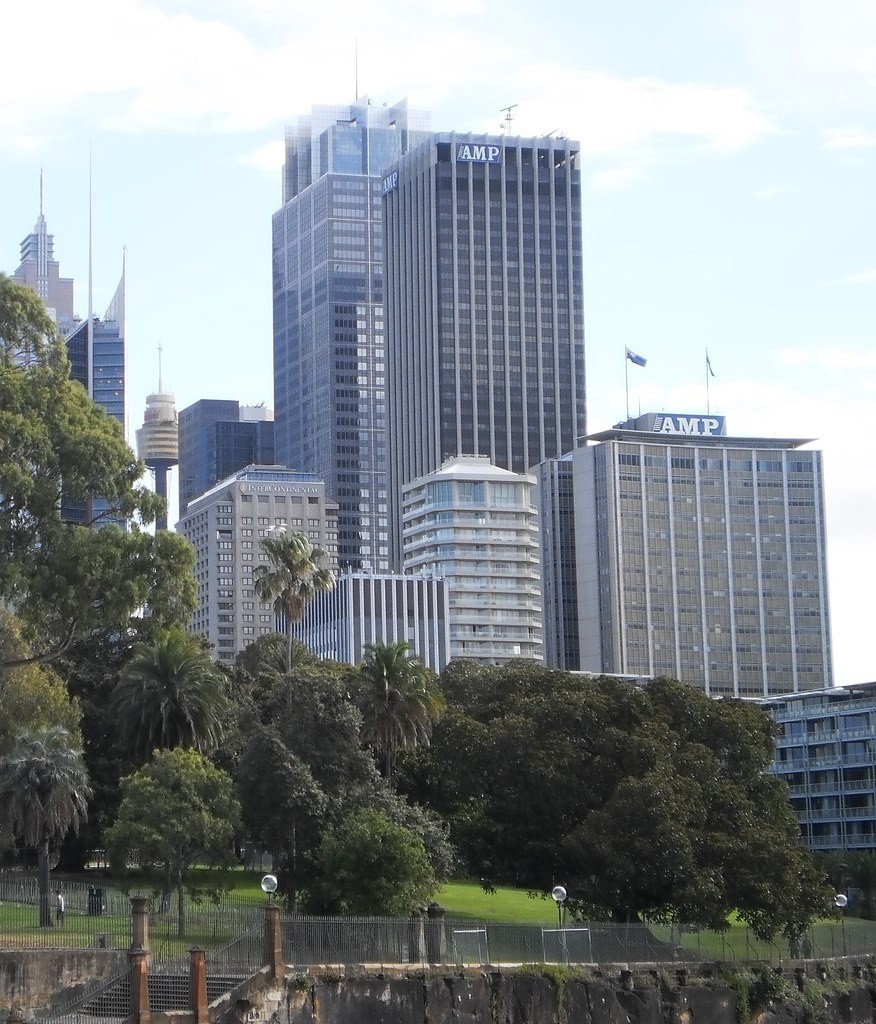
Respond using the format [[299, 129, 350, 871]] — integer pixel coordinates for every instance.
[[789, 934, 800, 959], [56, 890, 64, 927], [801, 935, 812, 959]]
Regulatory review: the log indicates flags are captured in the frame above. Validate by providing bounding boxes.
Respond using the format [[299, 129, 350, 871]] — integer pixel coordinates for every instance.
[[627, 348, 647, 367], [707, 354, 715, 377]]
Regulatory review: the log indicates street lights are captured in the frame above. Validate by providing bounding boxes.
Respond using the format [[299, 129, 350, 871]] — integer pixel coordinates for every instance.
[[553, 887, 567, 929], [261, 875, 277, 903], [835, 894, 847, 956]]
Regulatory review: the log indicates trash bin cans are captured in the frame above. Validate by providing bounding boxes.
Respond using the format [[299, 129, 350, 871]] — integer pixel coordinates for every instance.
[[88, 887, 108, 916]]
